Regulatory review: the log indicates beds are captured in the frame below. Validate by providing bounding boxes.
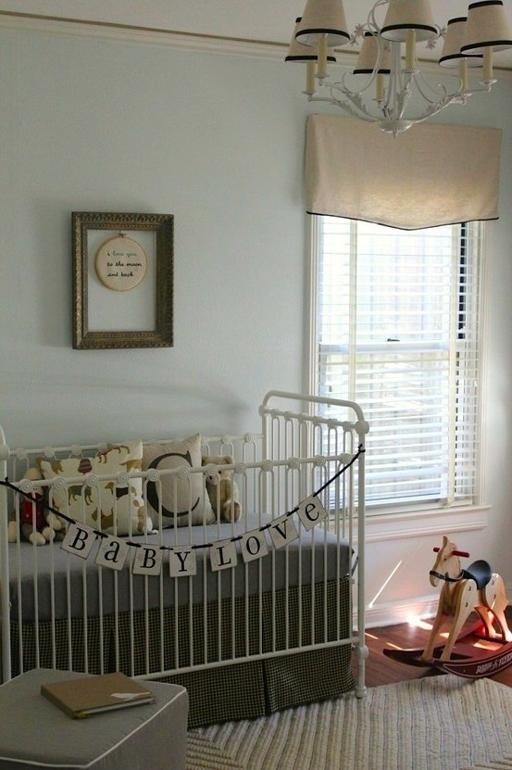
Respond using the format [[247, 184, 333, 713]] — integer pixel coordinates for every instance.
[[2, 384, 375, 769]]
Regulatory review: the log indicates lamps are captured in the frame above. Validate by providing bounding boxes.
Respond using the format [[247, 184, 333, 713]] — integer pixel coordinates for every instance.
[[280, 1, 511, 143]]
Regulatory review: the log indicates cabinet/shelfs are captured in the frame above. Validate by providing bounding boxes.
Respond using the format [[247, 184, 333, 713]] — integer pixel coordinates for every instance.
[[0, 664, 190, 768]]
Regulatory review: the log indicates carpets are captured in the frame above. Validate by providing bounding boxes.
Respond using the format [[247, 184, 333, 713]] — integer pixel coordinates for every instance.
[[190, 672, 511, 770]]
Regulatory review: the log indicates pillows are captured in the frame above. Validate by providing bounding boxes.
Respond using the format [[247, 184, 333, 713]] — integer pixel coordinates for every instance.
[[34, 437, 161, 543], [101, 430, 220, 537]]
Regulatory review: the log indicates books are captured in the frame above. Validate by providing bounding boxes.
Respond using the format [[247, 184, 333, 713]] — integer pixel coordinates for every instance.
[[41, 671, 157, 721]]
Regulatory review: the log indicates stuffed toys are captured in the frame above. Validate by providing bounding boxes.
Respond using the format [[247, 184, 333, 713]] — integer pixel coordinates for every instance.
[[201, 455, 243, 524], [7, 467, 63, 547]]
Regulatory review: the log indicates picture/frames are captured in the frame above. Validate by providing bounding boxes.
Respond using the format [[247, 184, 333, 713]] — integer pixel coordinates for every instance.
[[67, 202, 178, 355]]
